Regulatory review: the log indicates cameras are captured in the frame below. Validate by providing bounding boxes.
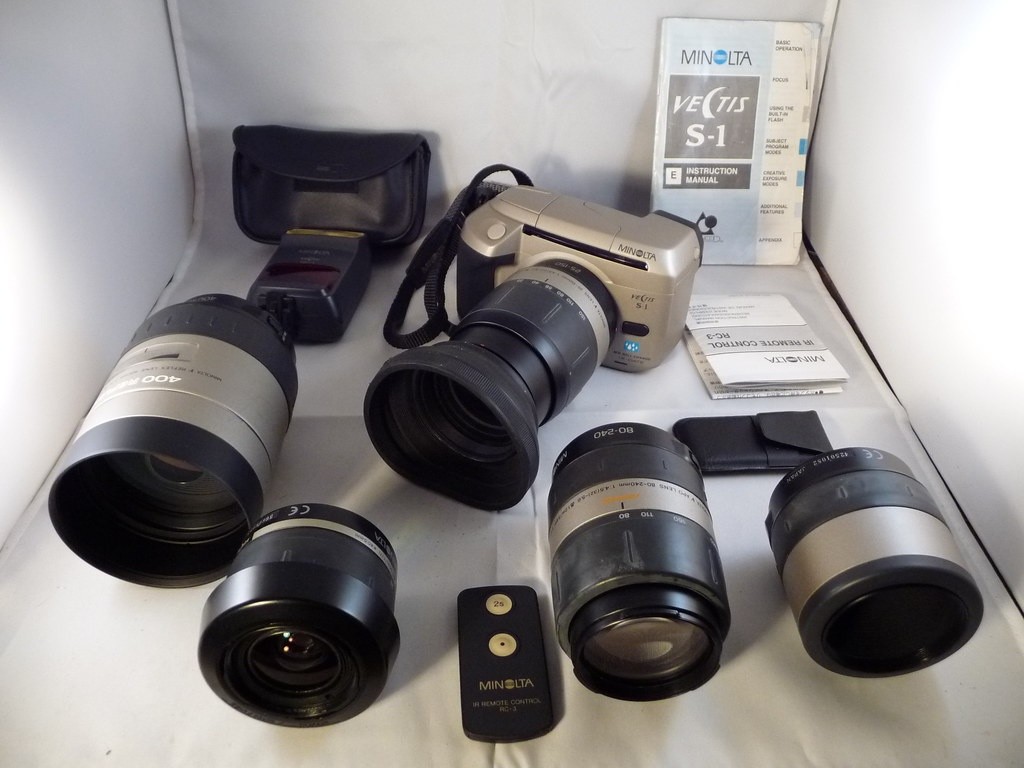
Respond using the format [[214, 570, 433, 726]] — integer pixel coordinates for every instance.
[[363, 175, 707, 514]]
